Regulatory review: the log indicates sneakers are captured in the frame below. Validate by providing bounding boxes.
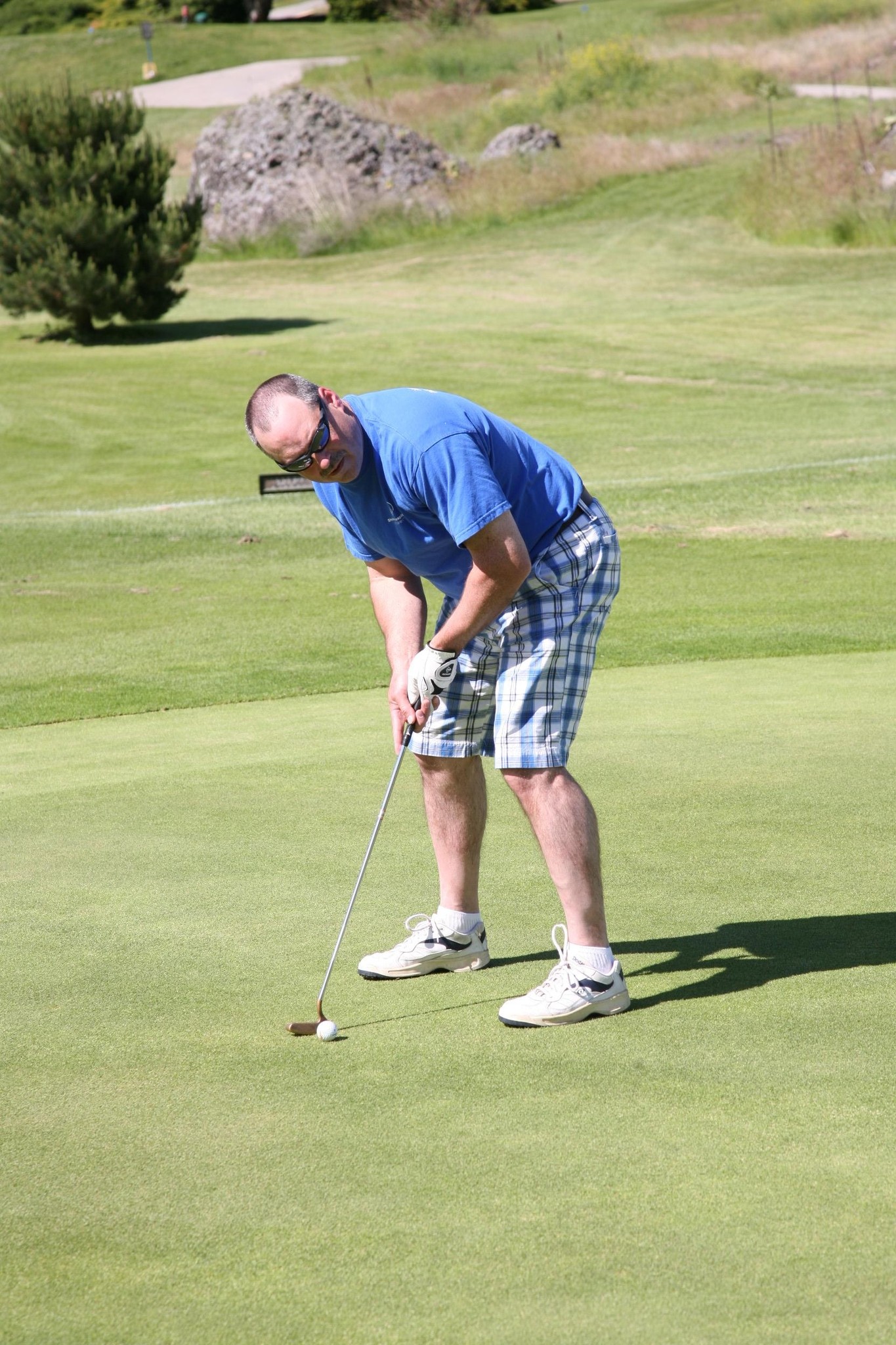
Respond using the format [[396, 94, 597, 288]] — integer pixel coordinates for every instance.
[[498, 959, 632, 1028], [358, 913, 491, 980]]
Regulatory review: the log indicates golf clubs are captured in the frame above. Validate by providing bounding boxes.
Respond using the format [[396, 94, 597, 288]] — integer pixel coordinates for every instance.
[[284, 700, 423, 1037]]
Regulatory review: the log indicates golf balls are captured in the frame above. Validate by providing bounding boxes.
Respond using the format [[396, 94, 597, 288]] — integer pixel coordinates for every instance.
[[317, 1021, 337, 1041]]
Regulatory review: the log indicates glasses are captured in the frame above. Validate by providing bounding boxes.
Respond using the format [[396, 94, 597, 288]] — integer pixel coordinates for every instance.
[[274, 397, 331, 472]]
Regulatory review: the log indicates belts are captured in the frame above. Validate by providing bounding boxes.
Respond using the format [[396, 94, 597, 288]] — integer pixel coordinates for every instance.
[[555, 486, 593, 539]]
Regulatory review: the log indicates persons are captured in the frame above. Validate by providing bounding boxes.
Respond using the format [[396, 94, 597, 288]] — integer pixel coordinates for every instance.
[[245, 374, 630, 1026]]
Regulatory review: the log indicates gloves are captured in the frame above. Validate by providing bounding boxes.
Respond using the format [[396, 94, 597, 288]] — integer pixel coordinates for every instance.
[[407, 640, 458, 717]]
